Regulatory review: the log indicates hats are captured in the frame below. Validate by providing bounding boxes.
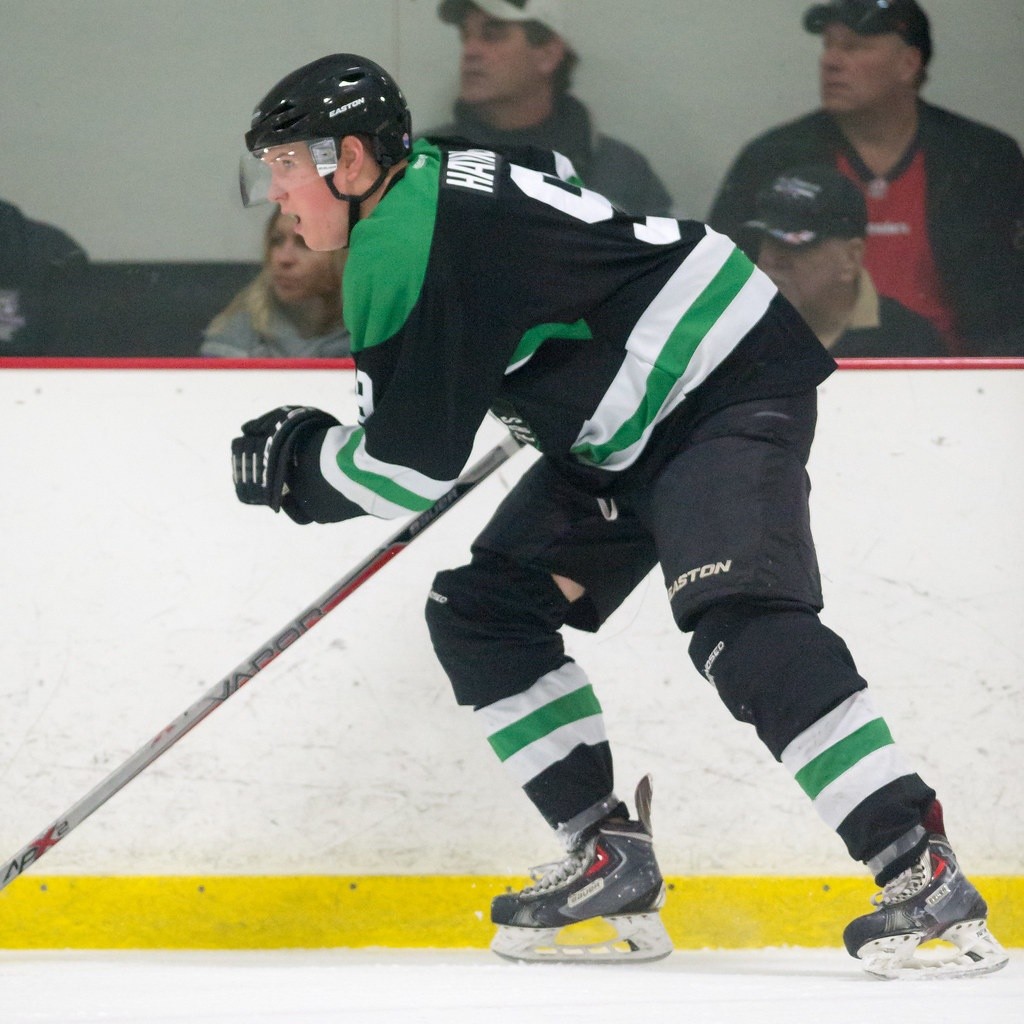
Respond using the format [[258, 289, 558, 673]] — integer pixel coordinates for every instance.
[[805, 0, 930, 48], [438, 0, 582, 61], [739, 163, 866, 250]]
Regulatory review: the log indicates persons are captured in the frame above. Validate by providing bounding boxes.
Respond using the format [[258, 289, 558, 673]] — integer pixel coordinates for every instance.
[[704, 0, 1024, 360], [226, 53, 1011, 978], [736, 161, 955, 362], [418, 1, 677, 230], [0, 193, 97, 365], [196, 205, 358, 362]]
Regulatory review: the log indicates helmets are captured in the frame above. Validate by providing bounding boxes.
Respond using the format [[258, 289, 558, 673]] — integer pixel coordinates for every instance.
[[244, 53, 411, 180]]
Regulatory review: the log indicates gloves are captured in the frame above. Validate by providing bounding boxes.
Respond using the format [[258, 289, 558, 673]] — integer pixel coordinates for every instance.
[[231, 404, 343, 526]]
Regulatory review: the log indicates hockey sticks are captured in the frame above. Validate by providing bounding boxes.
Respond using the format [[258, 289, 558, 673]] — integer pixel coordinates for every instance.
[[0, 424, 528, 889]]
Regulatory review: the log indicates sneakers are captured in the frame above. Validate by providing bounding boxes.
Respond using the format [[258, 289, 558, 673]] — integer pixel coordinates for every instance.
[[842, 823, 1010, 983], [490, 773, 674, 964]]
